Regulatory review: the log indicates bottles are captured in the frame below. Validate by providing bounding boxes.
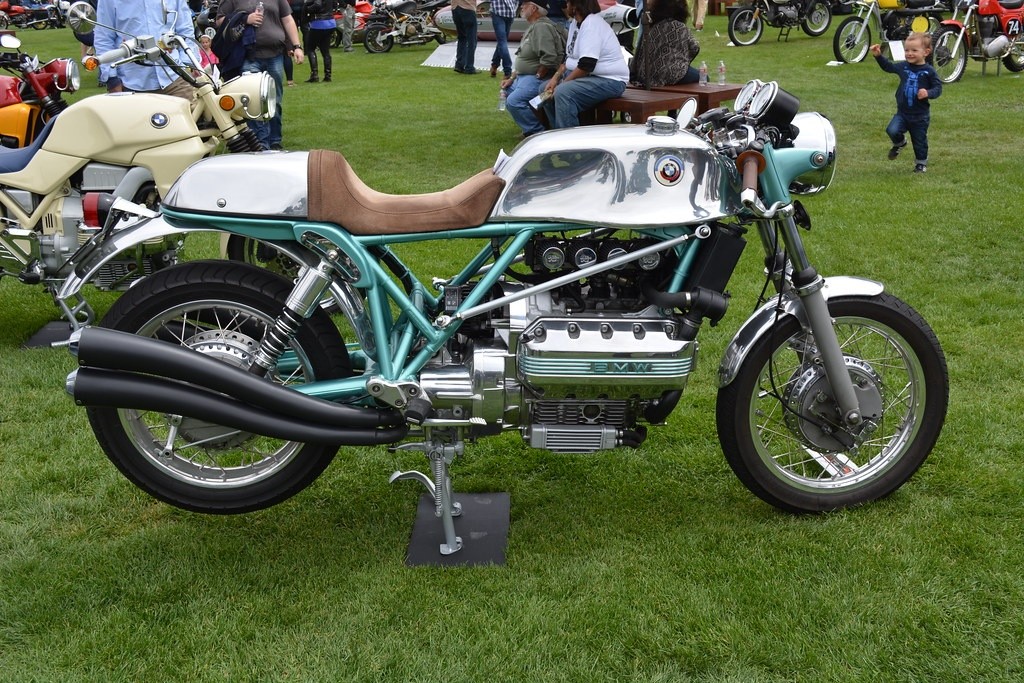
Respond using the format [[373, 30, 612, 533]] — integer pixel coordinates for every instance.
[[718, 60, 726, 86], [498, 86, 507, 111], [698, 61, 708, 86], [250, 2, 265, 27]]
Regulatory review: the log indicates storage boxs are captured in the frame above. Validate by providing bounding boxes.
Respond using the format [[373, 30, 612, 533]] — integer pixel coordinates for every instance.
[[0, 2, 10, 11], [8, 5, 25, 16]]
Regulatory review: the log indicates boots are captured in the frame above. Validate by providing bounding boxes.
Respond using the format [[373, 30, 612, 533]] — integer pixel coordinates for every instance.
[[305, 57, 319, 82], [321, 56, 331, 82]]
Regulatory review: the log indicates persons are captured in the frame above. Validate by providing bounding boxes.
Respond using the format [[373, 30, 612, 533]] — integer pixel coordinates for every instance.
[[501, 0, 629, 137], [216, 0, 304, 150], [870, 33, 942, 173], [451, 0, 483, 75], [489, 0, 519, 80], [284, 0, 356, 86], [630, 0, 709, 119], [198, 35, 218, 69], [80, 44, 94, 59], [93, 0, 202, 104]]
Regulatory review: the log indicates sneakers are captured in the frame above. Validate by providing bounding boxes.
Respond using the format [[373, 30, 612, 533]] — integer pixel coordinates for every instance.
[[913, 163, 927, 173], [888, 141, 907, 160]]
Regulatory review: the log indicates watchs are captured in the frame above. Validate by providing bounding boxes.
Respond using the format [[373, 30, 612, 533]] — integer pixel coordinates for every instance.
[[294, 44, 303, 50]]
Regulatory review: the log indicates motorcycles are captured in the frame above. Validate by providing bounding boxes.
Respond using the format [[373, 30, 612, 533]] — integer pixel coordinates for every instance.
[[1, 0, 277, 331], [728, 0, 1024, 84], [52, 78, 950, 558], [329, 0, 447, 54]]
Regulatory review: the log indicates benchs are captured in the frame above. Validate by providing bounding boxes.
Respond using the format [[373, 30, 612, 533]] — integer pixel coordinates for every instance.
[[528, 87, 699, 131], [626, 80, 745, 115]]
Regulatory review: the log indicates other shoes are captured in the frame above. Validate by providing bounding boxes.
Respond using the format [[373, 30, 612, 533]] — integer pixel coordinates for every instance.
[[453, 67, 465, 74], [288, 83, 297, 86], [500, 75, 510, 80], [465, 70, 482, 74], [270, 143, 283, 151], [490, 67, 497, 75]]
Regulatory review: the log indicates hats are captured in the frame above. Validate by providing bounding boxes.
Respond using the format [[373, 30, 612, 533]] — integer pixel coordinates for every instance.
[[529, 0, 552, 14]]
